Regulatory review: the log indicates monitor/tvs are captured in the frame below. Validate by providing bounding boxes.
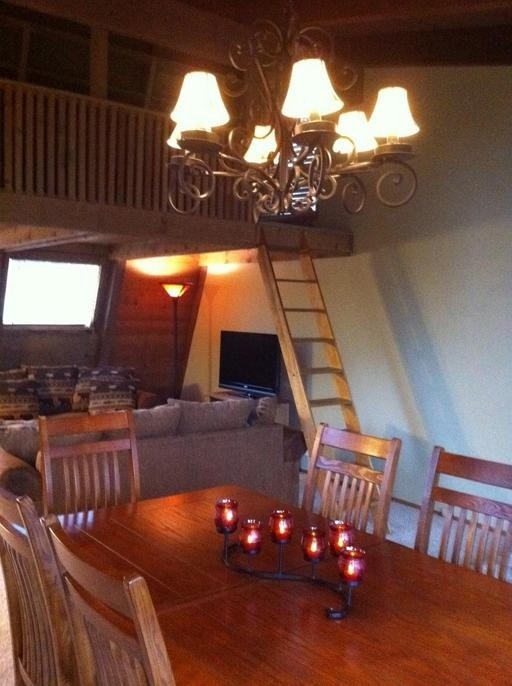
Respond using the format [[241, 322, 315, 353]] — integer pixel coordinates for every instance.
[[219, 331, 281, 399]]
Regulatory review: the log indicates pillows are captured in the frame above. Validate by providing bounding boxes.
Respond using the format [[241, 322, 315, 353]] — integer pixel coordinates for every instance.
[[0, 395, 287, 466], [0, 364, 139, 417]]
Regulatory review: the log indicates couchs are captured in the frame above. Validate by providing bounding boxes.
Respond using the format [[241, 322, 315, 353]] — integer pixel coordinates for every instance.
[[1, 424, 310, 515], [0, 364, 168, 425]]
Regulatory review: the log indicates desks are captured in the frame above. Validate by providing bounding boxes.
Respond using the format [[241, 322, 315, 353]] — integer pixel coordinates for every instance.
[[33, 482, 511, 684]]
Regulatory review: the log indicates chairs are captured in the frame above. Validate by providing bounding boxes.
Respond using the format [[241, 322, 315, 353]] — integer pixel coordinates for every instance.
[[38, 512, 177, 686], [302, 423, 401, 538], [414, 442, 512, 582], [0, 488, 78, 686], [38, 409, 141, 515]]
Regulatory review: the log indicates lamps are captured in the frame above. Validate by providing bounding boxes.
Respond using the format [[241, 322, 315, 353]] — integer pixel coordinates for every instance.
[[214, 497, 380, 619], [158, 0, 424, 225], [162, 282, 192, 398]]
